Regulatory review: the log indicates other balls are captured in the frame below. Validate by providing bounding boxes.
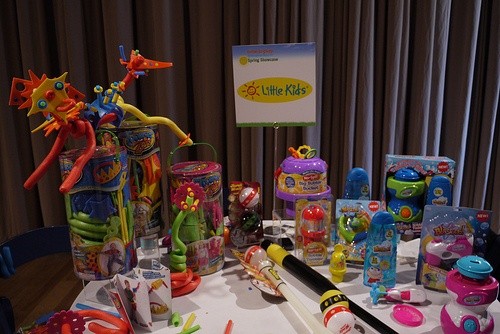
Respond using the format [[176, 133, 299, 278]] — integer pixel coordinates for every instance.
[[239, 188, 259, 208], [322, 305, 355, 334], [319, 289, 349, 314], [240, 212, 261, 231]]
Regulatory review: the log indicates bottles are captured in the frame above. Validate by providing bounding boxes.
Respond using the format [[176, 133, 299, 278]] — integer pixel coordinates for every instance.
[[440, 255, 499, 334], [342, 168, 370, 201], [362, 211, 398, 288]]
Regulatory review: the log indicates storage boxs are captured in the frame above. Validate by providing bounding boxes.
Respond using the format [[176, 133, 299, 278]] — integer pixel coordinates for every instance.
[[380, 154, 456, 235]]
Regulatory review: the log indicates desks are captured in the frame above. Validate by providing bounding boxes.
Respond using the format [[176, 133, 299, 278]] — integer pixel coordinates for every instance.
[[69, 220, 500, 334]]
